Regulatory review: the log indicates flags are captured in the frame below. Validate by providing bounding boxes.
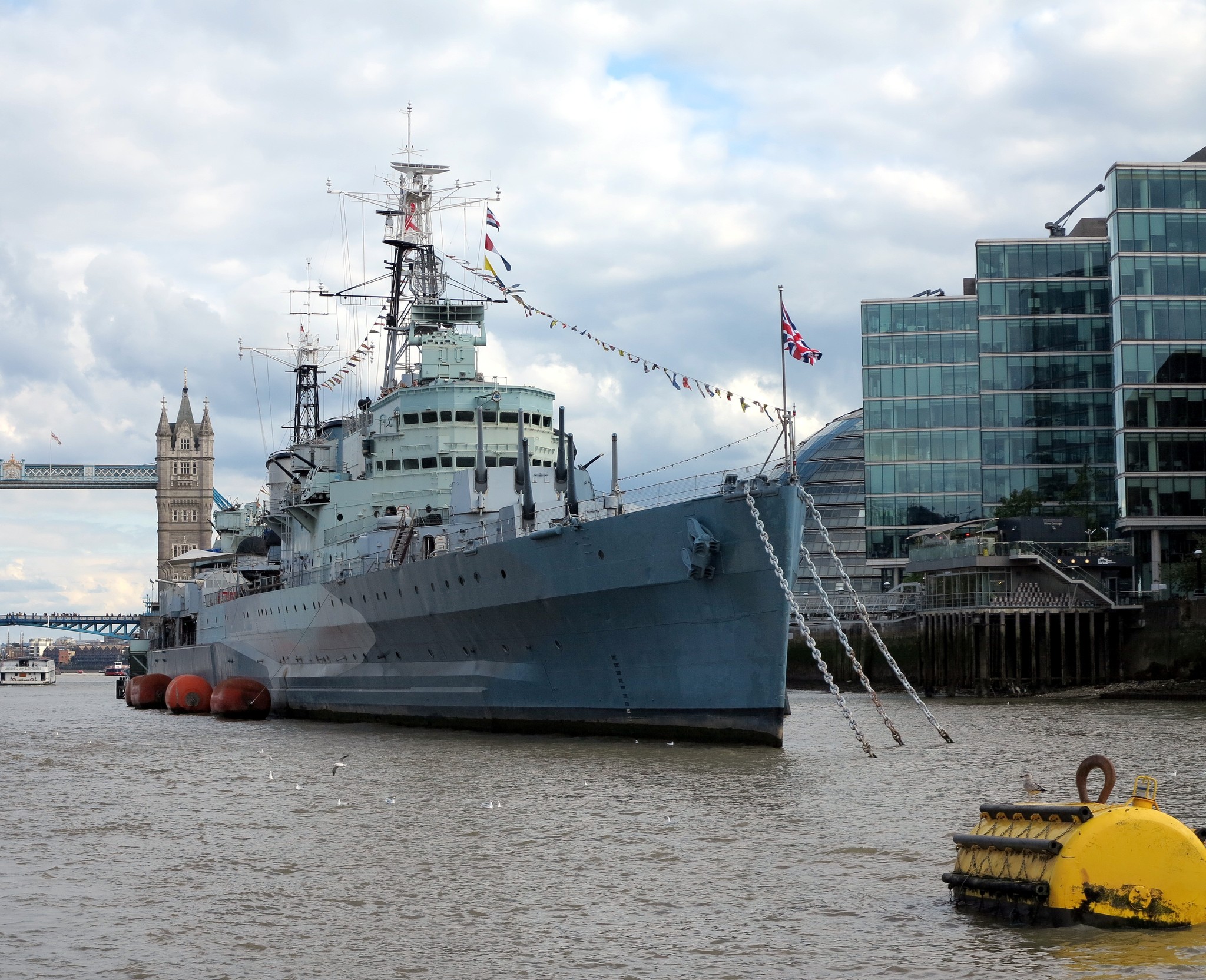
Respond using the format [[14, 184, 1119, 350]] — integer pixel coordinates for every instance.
[[51, 431, 62, 445], [486, 206, 500, 232], [782, 299, 823, 366], [483, 253, 506, 288], [402, 202, 419, 257], [316, 268, 409, 392], [446, 254, 785, 425], [485, 233, 511, 272]]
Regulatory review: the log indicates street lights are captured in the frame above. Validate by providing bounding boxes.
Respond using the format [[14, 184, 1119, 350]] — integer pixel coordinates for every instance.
[[838, 588, 845, 594], [883, 581, 891, 592], [1101, 527, 1110, 557], [1194, 550, 1206, 597], [1085, 528, 1097, 555]]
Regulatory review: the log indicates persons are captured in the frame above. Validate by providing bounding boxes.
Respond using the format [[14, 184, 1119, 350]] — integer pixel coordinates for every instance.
[[384, 509, 394, 516], [377, 380, 418, 400]]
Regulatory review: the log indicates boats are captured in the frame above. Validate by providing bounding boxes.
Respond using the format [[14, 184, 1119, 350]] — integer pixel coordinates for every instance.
[[0, 657, 57, 686], [129, 105, 810, 752], [105, 657, 128, 676]]
